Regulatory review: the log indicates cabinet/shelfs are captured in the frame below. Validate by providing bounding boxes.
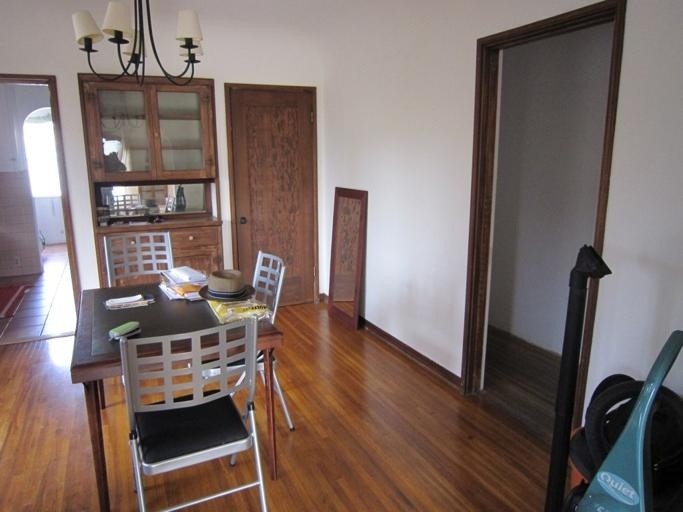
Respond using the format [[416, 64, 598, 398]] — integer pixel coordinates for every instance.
[[77, 73, 218, 183], [94, 225, 223, 287]]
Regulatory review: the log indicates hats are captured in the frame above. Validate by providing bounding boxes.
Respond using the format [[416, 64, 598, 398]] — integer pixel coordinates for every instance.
[[200, 269, 256, 303]]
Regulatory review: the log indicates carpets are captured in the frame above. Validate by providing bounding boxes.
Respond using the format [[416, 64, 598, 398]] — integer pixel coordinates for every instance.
[[0, 283, 34, 337]]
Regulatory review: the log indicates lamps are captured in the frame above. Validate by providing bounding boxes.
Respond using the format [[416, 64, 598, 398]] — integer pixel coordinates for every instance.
[[72, 0, 204, 87]]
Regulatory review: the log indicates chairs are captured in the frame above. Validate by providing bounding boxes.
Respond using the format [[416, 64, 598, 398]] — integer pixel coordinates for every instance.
[[119, 315, 269, 512], [112, 194, 140, 215], [164, 192, 175, 211], [103, 231, 177, 387], [187, 249, 296, 433]]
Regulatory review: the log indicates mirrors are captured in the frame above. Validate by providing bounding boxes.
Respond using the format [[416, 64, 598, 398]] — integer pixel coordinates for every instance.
[[95, 180, 213, 222], [328, 187, 368, 332]]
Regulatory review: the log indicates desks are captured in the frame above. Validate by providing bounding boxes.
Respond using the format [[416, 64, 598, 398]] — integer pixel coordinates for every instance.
[[71, 281, 286, 512]]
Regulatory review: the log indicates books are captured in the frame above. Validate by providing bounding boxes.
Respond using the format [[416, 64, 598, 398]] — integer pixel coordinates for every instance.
[[103, 265, 210, 311]]
